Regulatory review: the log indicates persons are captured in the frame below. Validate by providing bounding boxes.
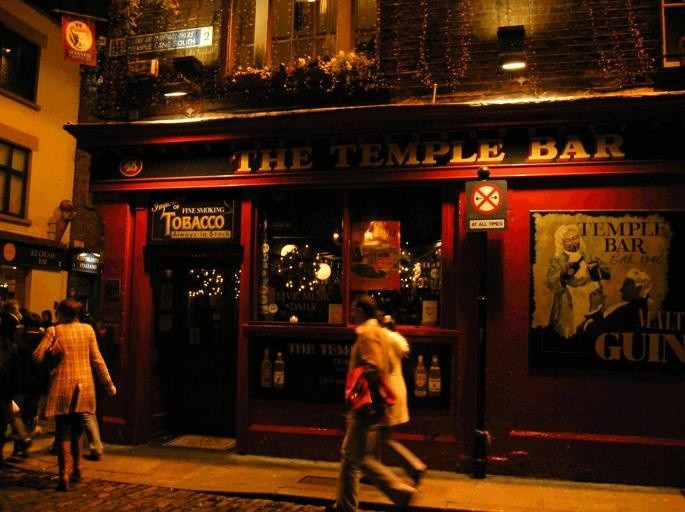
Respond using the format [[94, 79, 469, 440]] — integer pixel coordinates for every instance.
[[326, 294, 427, 512], [0, 279, 117, 490], [545, 223, 653, 348]]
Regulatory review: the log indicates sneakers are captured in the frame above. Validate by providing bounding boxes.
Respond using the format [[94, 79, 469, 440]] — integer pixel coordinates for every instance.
[[14, 435, 35, 452]]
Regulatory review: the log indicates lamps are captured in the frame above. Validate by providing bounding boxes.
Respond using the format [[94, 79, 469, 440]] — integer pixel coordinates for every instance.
[[160, 56, 203, 98], [497, 25, 530, 73]]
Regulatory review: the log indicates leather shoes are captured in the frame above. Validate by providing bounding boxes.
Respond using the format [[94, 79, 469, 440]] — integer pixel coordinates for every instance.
[[413, 467, 431, 489], [358, 477, 380, 487], [325, 503, 357, 512], [395, 490, 415, 510]]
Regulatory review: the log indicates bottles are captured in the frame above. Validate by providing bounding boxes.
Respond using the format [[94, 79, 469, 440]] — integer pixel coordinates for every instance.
[[414, 352, 442, 403], [259, 341, 285, 391], [561, 255, 585, 287], [417, 252, 439, 293]]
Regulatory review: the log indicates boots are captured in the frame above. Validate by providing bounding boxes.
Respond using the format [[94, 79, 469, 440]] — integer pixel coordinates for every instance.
[[59, 439, 71, 492], [71, 440, 84, 477]]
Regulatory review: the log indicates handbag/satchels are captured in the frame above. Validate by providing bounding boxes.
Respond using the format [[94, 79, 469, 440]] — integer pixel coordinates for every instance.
[[47, 326, 65, 362]]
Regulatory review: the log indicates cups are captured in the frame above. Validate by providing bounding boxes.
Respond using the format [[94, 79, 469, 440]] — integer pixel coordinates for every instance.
[[587, 261, 604, 281]]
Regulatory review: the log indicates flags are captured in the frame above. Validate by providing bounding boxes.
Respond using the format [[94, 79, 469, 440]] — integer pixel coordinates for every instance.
[[61, 15, 97, 66]]
